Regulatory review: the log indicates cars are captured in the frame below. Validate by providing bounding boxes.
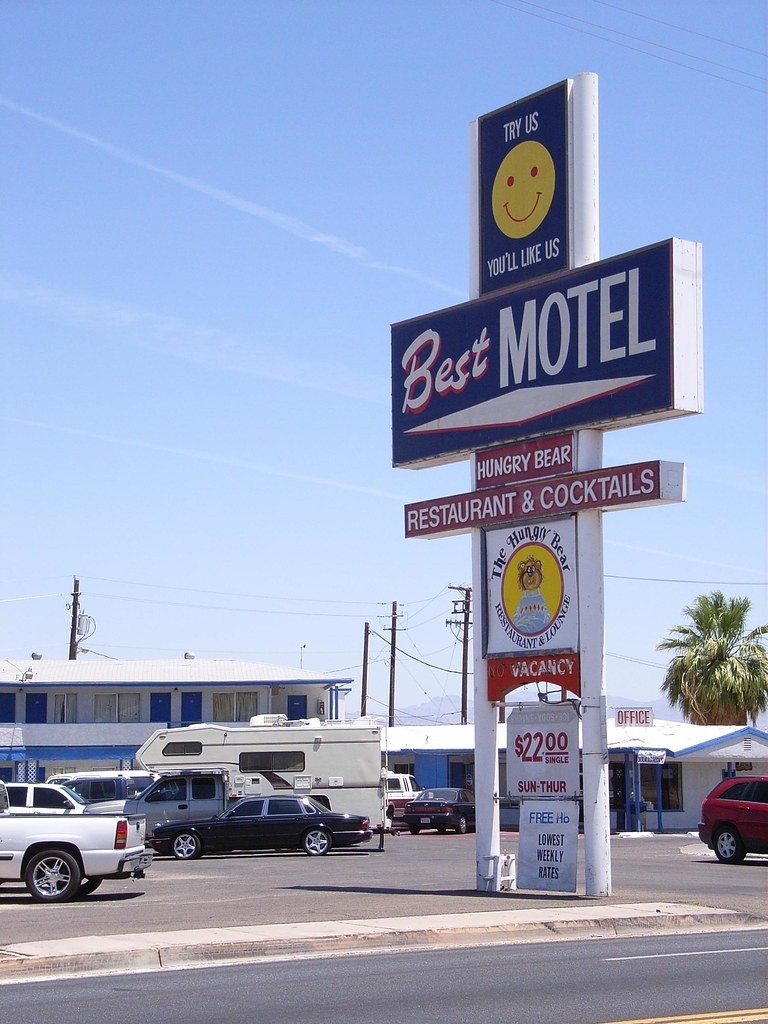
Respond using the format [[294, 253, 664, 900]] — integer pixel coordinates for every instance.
[[402, 787, 476, 835], [148, 792, 374, 861]]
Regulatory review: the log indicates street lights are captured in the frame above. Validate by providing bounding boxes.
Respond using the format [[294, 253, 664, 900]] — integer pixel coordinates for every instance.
[[300, 644, 307, 669]]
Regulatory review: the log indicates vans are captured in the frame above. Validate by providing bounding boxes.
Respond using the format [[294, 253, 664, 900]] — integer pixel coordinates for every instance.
[[44, 768, 160, 802]]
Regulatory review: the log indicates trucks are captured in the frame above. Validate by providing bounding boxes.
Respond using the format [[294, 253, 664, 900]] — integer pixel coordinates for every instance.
[[84, 714, 393, 845]]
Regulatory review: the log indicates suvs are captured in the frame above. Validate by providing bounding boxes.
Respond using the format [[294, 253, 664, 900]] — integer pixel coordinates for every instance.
[[381, 769, 433, 823], [5, 780, 93, 816], [697, 774, 768, 864]]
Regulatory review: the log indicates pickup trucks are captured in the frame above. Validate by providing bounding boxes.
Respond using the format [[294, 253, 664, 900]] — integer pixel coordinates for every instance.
[[0, 779, 153, 904]]
